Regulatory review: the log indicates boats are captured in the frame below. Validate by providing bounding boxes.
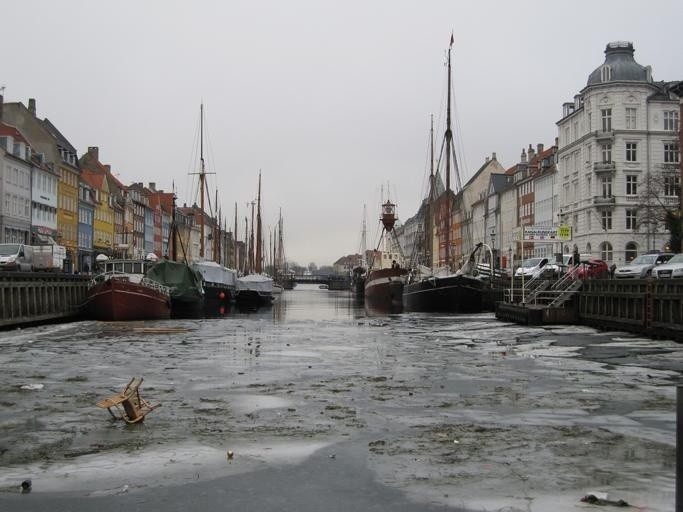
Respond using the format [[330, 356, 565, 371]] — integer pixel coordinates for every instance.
[[87, 243, 170, 320]]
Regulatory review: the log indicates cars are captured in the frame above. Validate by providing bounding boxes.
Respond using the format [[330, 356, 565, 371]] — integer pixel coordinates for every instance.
[[567, 259, 607, 280], [651, 253, 683, 279], [614, 252, 675, 279]]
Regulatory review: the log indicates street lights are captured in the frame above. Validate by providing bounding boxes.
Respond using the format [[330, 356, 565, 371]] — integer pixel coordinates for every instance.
[[490, 229, 496, 280], [557, 209, 566, 275], [652, 221, 658, 252]]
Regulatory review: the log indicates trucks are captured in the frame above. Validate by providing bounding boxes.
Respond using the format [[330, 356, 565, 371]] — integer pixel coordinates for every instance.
[[543, 254, 602, 277], [33, 245, 66, 271]]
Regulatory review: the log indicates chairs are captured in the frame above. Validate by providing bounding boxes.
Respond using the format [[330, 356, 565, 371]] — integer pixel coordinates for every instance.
[[96, 377, 162, 424]]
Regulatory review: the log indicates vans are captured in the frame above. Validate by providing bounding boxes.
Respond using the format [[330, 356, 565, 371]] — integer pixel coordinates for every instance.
[[0, 243, 33, 272], [514, 258, 557, 280]]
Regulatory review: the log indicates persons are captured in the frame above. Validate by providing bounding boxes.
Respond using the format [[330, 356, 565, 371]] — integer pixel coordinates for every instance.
[[610, 263, 616, 279], [392, 260, 400, 269], [493, 250, 500, 269], [574, 250, 580, 266]]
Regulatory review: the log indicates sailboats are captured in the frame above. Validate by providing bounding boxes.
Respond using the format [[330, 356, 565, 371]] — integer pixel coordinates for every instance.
[[401, 27, 490, 313], [142, 97, 296, 314], [364, 180, 410, 303], [327, 203, 370, 293]]
